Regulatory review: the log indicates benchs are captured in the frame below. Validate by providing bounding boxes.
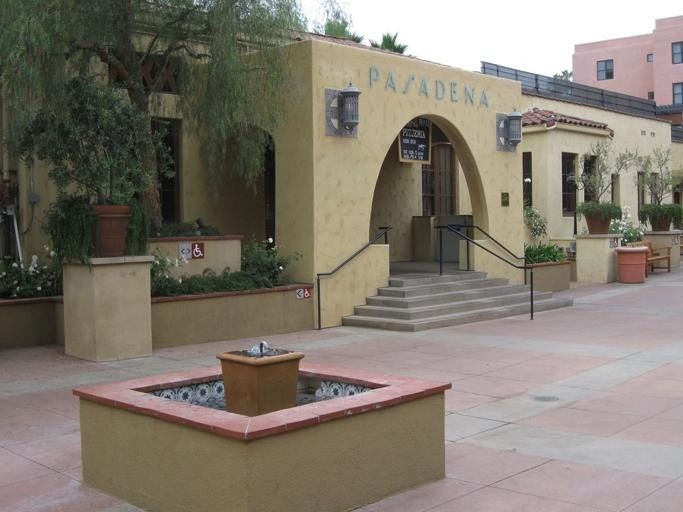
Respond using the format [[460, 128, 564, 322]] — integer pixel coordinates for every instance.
[[626, 241, 672, 277]]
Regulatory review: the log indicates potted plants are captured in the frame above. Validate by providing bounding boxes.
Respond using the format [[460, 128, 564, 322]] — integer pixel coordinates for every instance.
[[671, 203, 683, 229], [576, 201, 620, 233], [638, 202, 676, 231], [17, 72, 154, 281]]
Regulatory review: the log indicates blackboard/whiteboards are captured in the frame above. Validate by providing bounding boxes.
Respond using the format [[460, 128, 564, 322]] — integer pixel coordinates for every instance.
[[400, 117, 432, 165]]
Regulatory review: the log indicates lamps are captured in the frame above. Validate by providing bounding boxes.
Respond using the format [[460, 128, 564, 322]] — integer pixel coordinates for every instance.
[[504, 108, 522, 144], [338, 82, 361, 130]]
